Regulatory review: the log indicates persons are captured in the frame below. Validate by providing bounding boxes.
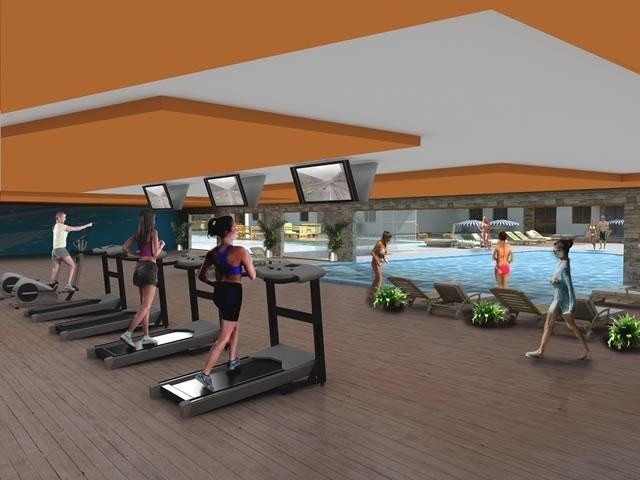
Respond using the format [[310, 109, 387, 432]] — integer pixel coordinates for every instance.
[[370, 230, 392, 295], [120, 210, 165, 348], [596, 214, 609, 249], [47, 210, 94, 291], [492, 231, 513, 288], [588, 217, 597, 251], [478, 216, 490, 247], [194, 215, 257, 393], [524, 238, 590, 360]]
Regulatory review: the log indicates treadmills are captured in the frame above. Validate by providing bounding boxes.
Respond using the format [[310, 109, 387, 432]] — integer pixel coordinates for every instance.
[[22, 245, 128, 322], [49, 250, 169, 341], [85, 253, 229, 368], [148, 258, 328, 420]]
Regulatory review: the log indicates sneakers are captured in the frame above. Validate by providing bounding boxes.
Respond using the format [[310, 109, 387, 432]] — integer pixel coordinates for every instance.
[[526, 350, 544, 359], [120, 331, 136, 349], [228, 357, 240, 370], [577, 351, 591, 359], [195, 373, 214, 391], [142, 337, 158, 345], [65, 284, 76, 291]]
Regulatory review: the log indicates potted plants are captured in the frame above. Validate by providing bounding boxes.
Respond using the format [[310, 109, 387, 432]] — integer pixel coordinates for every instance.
[[319, 217, 350, 262], [257, 219, 288, 258], [170, 222, 195, 251]]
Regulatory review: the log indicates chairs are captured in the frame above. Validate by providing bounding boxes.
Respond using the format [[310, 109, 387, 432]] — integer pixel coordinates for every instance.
[[489, 288, 564, 328], [387, 276, 441, 306], [458, 230, 552, 249], [427, 283, 493, 320], [234, 222, 322, 240], [574, 296, 626, 341]]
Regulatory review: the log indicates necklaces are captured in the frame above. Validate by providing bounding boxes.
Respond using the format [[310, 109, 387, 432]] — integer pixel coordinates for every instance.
[[560, 258, 570, 260]]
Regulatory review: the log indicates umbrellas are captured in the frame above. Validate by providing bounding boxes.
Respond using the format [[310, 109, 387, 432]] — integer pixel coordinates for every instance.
[[489, 219, 520, 227], [456, 219, 481, 227]]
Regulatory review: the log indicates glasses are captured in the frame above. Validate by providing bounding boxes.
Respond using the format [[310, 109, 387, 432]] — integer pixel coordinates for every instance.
[[553, 249, 562, 254]]
